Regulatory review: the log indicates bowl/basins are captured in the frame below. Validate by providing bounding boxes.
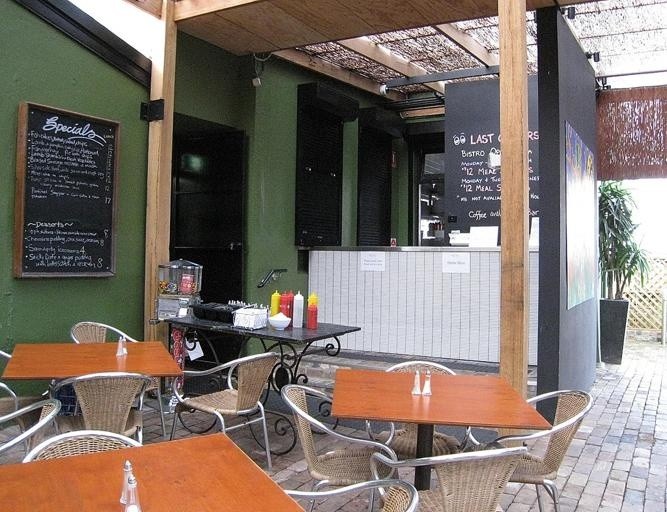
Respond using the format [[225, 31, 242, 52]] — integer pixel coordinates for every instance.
[[269, 318, 291, 331]]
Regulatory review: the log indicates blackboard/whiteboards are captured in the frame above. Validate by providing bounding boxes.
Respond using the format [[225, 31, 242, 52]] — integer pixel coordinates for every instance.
[[446, 73, 542, 232], [12, 101, 122, 279]]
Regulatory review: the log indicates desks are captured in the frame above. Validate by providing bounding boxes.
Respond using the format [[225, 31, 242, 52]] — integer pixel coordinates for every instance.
[[148, 311, 362, 457]]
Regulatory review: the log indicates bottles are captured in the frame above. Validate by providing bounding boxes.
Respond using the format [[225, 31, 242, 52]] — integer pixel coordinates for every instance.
[[268, 291, 319, 328], [114, 333, 128, 357], [422, 368, 433, 396], [410, 369, 422, 396], [116, 457, 142, 511]]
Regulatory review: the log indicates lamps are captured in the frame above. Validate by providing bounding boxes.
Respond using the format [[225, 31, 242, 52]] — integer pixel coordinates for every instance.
[[561, 7, 607, 85]]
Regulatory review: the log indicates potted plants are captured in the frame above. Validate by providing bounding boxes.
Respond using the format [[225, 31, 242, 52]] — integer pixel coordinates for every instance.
[[598, 177, 648, 363]]
